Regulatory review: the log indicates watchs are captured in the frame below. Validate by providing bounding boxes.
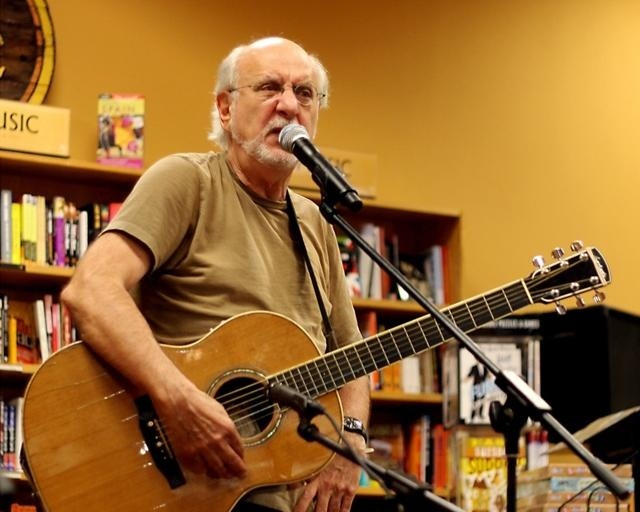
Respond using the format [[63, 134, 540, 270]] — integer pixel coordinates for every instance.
[[344, 414, 370, 446]]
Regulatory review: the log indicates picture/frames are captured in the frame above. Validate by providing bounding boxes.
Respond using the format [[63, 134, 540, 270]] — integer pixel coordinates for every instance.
[[458, 334, 542, 431]]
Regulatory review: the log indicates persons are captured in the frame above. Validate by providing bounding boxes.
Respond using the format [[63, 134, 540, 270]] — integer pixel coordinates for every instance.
[[458, 347, 491, 423], [60, 37, 370, 512]]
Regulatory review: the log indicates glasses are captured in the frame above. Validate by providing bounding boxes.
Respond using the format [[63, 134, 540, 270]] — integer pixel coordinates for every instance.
[[231, 82, 325, 104]]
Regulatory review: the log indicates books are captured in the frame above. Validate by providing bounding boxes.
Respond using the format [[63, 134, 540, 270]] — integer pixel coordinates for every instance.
[[375, 328, 446, 390], [368, 418, 449, 493], [1, 396, 30, 473], [0, 190, 130, 269], [340, 222, 454, 302], [515, 462, 634, 512], [1, 290, 78, 367], [96, 91, 145, 164]]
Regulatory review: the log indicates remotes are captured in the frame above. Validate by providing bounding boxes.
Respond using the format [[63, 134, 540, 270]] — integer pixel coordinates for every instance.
[[278, 123, 363, 214]]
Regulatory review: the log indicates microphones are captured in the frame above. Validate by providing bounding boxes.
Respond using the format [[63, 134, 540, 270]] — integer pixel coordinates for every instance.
[[264, 378, 310, 415]]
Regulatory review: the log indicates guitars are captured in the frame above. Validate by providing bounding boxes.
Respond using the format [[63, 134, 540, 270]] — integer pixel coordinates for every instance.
[[23, 241, 611, 512]]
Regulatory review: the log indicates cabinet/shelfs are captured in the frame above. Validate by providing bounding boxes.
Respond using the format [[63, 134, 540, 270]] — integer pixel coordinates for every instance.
[[0, 150, 464, 511]]
